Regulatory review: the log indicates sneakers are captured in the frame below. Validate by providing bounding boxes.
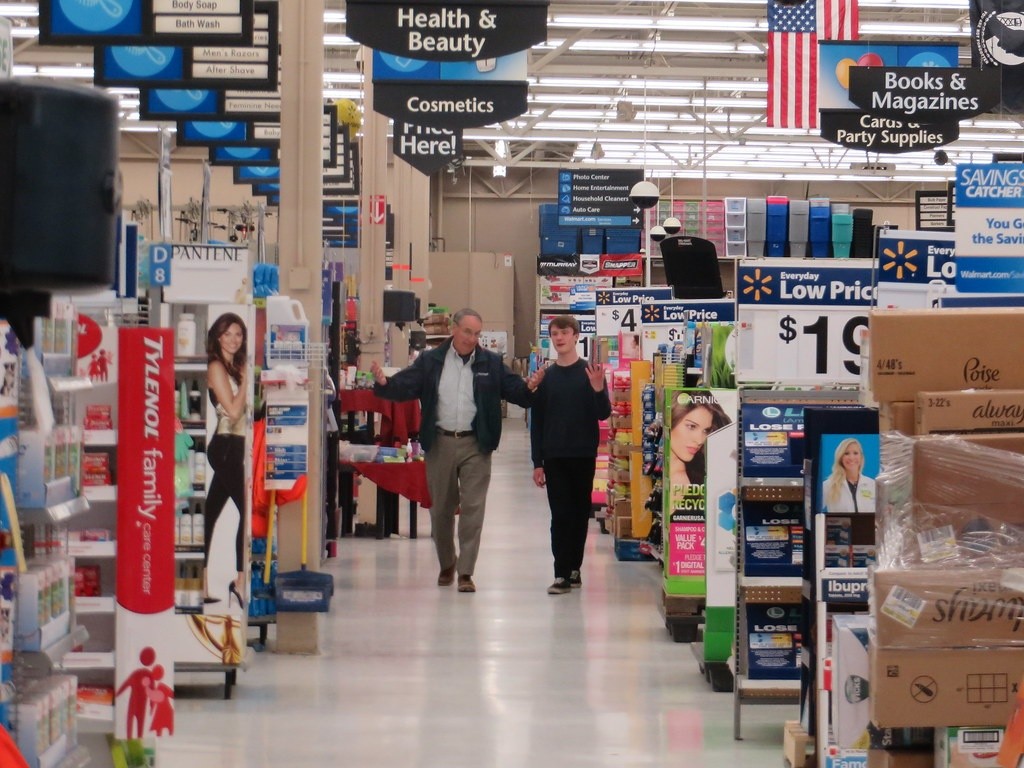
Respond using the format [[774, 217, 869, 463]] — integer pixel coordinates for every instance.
[[570, 570, 581, 587], [438, 556, 457, 586], [457, 574, 475, 592], [547, 577, 571, 594]]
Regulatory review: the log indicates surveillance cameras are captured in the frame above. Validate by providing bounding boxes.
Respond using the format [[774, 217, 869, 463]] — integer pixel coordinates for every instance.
[[356, 49, 364, 69], [640, 249, 646, 254], [650, 226, 666, 241], [663, 218, 682, 234], [629, 181, 660, 209]]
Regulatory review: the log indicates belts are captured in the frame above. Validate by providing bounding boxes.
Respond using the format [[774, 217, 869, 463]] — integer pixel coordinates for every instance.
[[436, 426, 475, 439]]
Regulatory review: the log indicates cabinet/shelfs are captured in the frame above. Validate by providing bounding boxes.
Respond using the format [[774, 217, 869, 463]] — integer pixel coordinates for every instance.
[[536, 257, 647, 360], [15, 239, 309, 768]]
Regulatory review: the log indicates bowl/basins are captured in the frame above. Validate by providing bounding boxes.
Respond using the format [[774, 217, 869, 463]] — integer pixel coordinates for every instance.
[[347, 444, 397, 463]]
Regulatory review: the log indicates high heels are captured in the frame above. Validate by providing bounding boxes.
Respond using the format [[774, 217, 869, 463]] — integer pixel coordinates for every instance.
[[228, 582, 243, 609], [203, 596, 220, 603]]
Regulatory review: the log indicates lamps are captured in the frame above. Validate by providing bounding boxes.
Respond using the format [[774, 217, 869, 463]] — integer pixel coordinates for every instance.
[[629, 75, 660, 210], [592, 137, 604, 159], [663, 164, 682, 235], [618, 94, 637, 122], [648, 172, 667, 242]]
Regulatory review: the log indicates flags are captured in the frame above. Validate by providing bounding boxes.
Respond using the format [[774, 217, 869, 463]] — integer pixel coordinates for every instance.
[[766, 0, 859, 130]]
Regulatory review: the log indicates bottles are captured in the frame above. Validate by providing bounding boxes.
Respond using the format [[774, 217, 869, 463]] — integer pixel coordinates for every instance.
[[174, 313, 205, 609]]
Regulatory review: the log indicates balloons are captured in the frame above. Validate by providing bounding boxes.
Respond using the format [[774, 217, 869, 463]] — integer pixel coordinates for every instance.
[[332, 99, 361, 140], [835, 53, 884, 92]]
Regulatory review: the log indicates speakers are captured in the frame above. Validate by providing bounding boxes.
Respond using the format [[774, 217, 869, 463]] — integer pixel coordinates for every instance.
[[384, 290, 427, 350]]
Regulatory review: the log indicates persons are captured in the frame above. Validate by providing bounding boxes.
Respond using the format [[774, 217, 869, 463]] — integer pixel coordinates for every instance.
[[323, 365, 338, 538], [530, 316, 612, 594], [206, 313, 246, 611], [631, 335, 640, 358], [822, 438, 876, 513], [369, 309, 545, 592], [669, 390, 732, 523]]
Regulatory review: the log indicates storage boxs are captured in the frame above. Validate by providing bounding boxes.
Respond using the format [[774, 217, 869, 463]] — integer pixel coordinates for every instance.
[[540, 202, 642, 358], [649, 164, 1024, 768]]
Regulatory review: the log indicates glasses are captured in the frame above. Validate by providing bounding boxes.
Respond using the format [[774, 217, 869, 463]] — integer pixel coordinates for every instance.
[[453, 321, 481, 339]]
